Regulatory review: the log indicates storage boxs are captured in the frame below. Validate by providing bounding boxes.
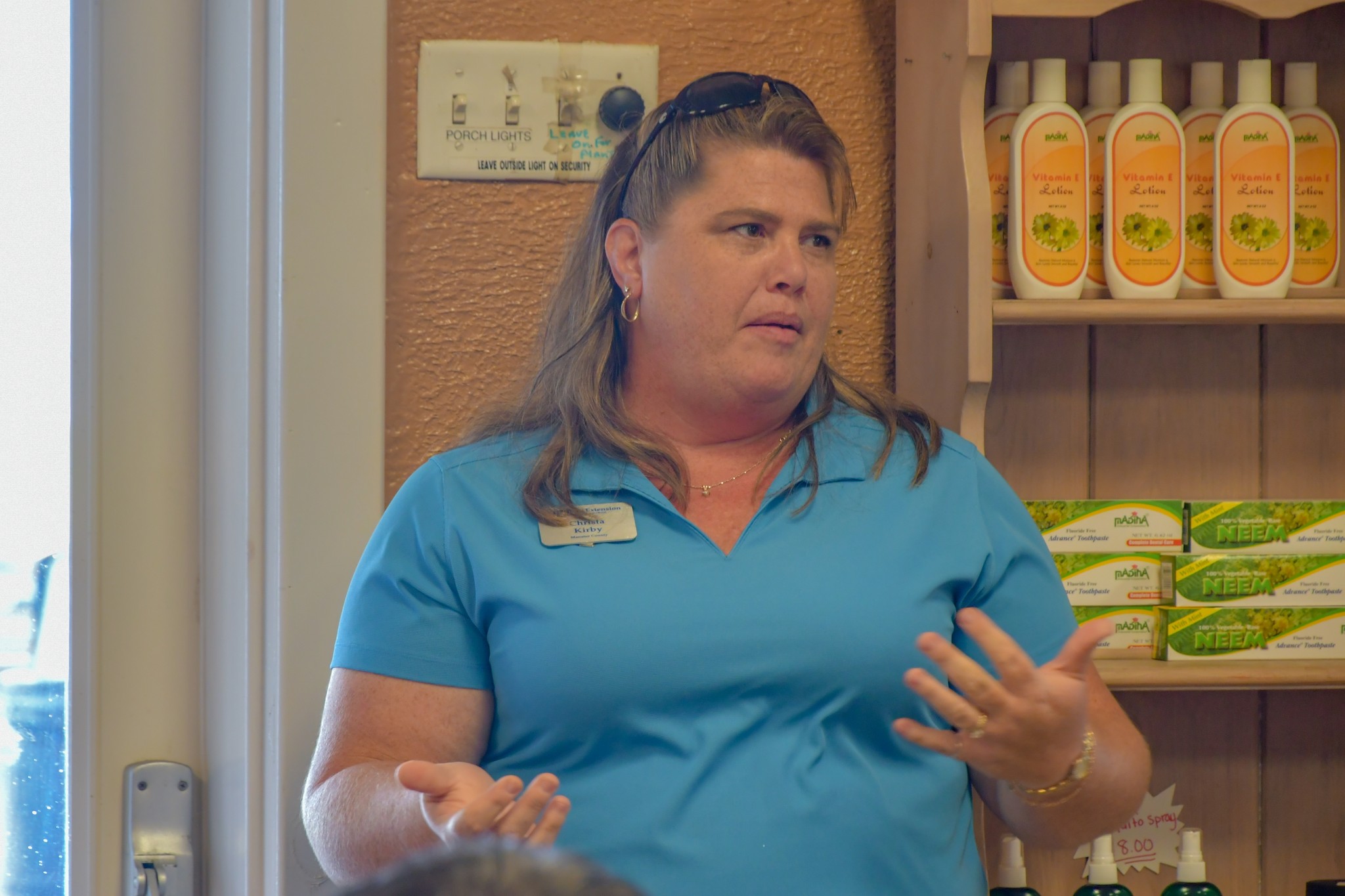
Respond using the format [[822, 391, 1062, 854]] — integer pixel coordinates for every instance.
[[1012, 498, 1345, 670]]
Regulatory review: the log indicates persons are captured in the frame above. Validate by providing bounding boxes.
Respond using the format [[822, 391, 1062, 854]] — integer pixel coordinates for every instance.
[[298, 75, 1158, 896]]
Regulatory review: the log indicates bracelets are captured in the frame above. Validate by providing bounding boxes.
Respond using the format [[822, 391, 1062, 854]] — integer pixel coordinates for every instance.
[[1005, 726, 1099, 809]]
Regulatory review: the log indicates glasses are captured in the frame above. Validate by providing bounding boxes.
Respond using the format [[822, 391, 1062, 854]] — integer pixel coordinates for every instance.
[[618, 72, 820, 220]]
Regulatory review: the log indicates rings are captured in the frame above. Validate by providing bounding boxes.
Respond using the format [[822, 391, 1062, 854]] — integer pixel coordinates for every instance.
[[964, 712, 993, 743]]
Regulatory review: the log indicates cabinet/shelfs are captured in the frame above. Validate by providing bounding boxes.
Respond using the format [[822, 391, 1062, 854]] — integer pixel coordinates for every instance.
[[883, 0, 1345, 896]]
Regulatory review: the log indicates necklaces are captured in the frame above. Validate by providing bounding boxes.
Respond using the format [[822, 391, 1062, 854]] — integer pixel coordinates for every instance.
[[680, 427, 796, 498]]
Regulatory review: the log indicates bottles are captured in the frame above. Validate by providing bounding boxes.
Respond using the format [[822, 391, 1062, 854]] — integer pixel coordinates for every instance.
[[1075, 61, 1122, 296], [1008, 57, 1092, 297], [1175, 60, 1235, 290], [1103, 57, 1186, 298], [1276, 63, 1342, 290], [1065, 831, 1135, 896], [1211, 59, 1297, 303], [990, 836, 1039, 896], [1161, 825, 1223, 896], [980, 57, 1038, 303]]
